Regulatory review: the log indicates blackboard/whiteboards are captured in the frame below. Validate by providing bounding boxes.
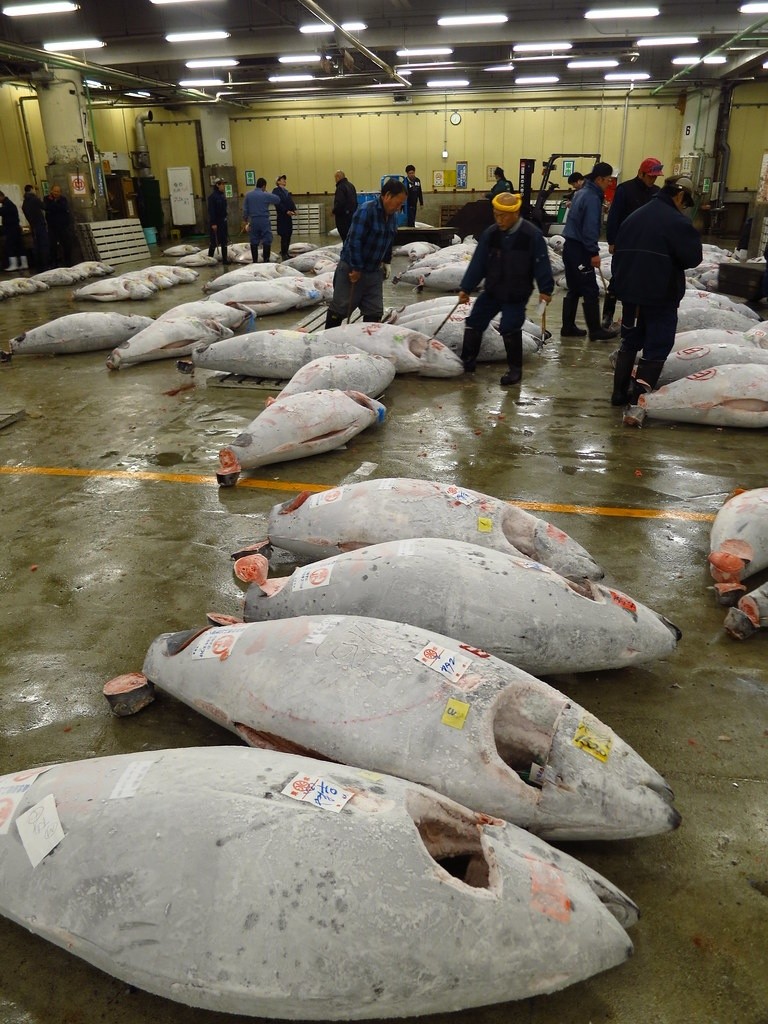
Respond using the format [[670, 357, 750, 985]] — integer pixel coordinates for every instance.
[[167, 167, 196, 224]]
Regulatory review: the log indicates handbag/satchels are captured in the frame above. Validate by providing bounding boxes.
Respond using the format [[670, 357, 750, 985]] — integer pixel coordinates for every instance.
[[556, 199, 570, 224]]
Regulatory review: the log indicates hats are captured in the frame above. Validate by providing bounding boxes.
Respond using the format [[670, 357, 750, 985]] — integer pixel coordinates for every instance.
[[664, 175, 696, 208], [639, 159, 664, 178], [215, 177, 227, 184], [275, 175, 286, 186]]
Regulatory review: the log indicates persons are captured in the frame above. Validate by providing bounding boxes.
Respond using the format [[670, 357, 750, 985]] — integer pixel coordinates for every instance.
[[0, 182, 76, 272], [610, 174, 703, 406], [602, 158, 664, 329], [330, 169, 358, 244], [565, 172, 584, 209], [242, 177, 280, 263], [397, 165, 423, 228], [458, 192, 555, 384], [325, 179, 407, 330], [481, 167, 514, 205], [561, 162, 618, 341], [207, 178, 233, 265], [272, 174, 298, 261]]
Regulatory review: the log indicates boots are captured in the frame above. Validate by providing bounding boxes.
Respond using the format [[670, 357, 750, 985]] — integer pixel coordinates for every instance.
[[610, 350, 635, 404], [561, 297, 587, 336], [281, 237, 296, 260], [500, 331, 524, 385], [600, 293, 617, 328], [362, 316, 379, 322], [222, 244, 232, 264], [208, 245, 215, 257], [4, 257, 19, 271], [636, 359, 666, 403], [250, 244, 258, 263], [580, 298, 617, 341], [460, 317, 482, 371], [325, 308, 345, 330], [19, 256, 29, 268], [263, 245, 271, 262]]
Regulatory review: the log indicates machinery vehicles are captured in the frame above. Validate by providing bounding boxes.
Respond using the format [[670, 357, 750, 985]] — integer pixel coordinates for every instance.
[[518, 152, 606, 240]]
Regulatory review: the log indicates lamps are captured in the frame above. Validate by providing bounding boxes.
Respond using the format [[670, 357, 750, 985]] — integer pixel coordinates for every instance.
[[1, 0, 768, 90]]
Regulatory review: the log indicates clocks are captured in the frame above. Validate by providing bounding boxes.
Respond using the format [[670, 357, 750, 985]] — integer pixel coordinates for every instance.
[[450, 112, 462, 126]]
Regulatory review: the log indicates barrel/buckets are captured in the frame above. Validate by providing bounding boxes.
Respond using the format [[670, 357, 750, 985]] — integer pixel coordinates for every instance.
[[143, 227, 158, 244]]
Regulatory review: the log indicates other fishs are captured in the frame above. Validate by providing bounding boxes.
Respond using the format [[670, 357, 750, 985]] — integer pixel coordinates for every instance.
[[101, 612, 682, 841], [231, 478, 606, 585], [0, 743, 640, 1021], [709, 488, 768, 642], [217, 388, 387, 488], [176, 328, 365, 378], [0, 241, 350, 370], [232, 537, 681, 675], [328, 217, 768, 429], [314, 322, 463, 378], [265, 353, 395, 408]]
